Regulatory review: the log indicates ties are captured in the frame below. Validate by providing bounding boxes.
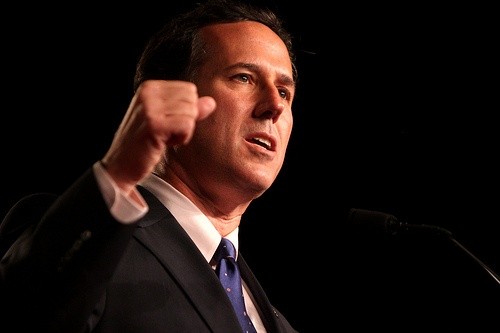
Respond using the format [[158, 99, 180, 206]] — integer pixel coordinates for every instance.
[[213, 237, 257, 333]]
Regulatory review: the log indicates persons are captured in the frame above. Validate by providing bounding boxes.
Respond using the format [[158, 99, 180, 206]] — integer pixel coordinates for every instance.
[[0, 0, 302, 333]]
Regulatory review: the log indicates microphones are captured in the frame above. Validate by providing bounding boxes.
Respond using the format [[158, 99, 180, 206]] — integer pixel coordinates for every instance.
[[349, 208, 452, 238]]
